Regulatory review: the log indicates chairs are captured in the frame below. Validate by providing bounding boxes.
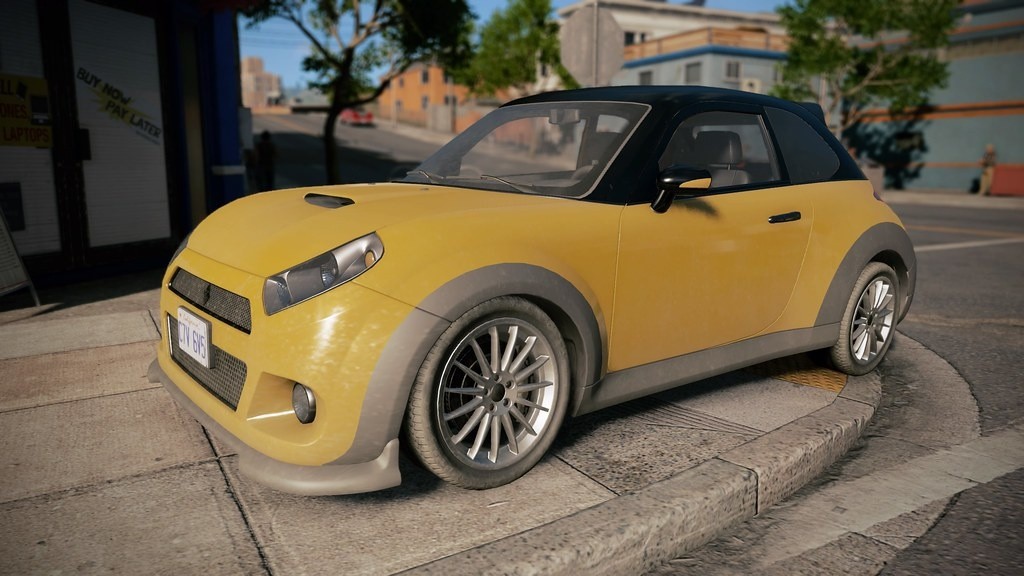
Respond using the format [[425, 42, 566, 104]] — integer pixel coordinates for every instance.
[[582, 130, 755, 187]]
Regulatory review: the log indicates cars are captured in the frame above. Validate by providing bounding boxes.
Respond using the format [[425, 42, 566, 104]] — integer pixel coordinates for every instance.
[[340, 110, 372, 126], [147, 86, 918, 495]]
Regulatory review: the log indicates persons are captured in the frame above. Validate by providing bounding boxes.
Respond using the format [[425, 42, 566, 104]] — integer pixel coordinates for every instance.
[[975, 143, 997, 196]]
